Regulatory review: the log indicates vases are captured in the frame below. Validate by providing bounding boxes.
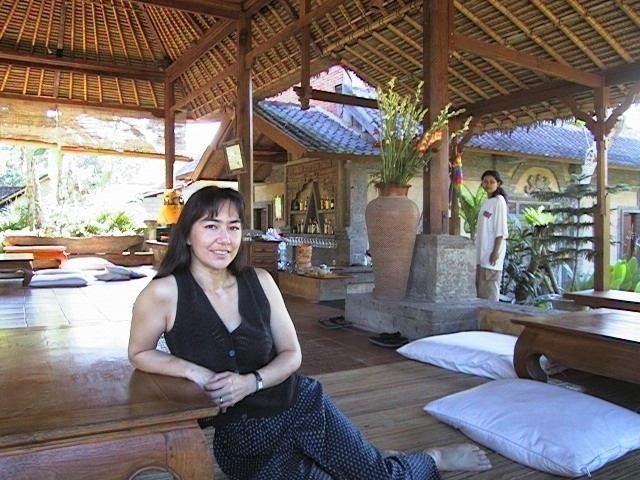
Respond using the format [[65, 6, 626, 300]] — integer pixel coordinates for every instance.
[[364, 181, 421, 302]]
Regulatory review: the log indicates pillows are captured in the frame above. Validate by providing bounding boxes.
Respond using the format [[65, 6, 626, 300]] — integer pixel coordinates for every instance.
[[395, 329, 569, 380], [423, 377, 640, 477], [28, 256, 147, 287]]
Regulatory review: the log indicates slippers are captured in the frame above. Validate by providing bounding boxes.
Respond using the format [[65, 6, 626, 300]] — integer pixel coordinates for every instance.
[[318, 316, 353, 330], [368, 332, 409, 348]]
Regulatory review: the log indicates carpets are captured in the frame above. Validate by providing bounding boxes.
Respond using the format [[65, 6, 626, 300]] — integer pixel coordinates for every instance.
[[199, 358, 640, 480]]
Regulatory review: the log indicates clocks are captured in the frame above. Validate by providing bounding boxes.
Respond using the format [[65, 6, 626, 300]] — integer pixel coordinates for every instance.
[[220, 136, 248, 177]]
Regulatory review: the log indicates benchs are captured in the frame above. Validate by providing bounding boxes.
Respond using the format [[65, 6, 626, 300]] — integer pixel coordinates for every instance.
[[562, 289, 640, 313], [6, 234, 144, 256]]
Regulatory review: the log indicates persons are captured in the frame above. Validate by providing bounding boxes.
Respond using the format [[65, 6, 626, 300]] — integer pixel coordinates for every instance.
[[473, 167, 510, 302], [126, 183, 494, 478]]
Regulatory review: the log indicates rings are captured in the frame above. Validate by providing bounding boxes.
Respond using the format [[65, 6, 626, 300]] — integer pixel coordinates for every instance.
[[217, 395, 225, 406]]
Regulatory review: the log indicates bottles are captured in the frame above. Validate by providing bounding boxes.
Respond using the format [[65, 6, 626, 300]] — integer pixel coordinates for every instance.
[[292, 194, 336, 235]]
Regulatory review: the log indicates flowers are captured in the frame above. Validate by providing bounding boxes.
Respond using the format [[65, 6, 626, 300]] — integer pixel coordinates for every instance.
[[364, 76, 474, 192]]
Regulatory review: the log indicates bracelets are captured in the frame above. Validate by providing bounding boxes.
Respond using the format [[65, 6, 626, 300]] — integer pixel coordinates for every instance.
[[251, 368, 264, 394]]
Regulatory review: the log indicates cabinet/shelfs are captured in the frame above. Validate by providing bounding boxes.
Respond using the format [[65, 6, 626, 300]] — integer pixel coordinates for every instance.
[[288, 182, 337, 239]]
